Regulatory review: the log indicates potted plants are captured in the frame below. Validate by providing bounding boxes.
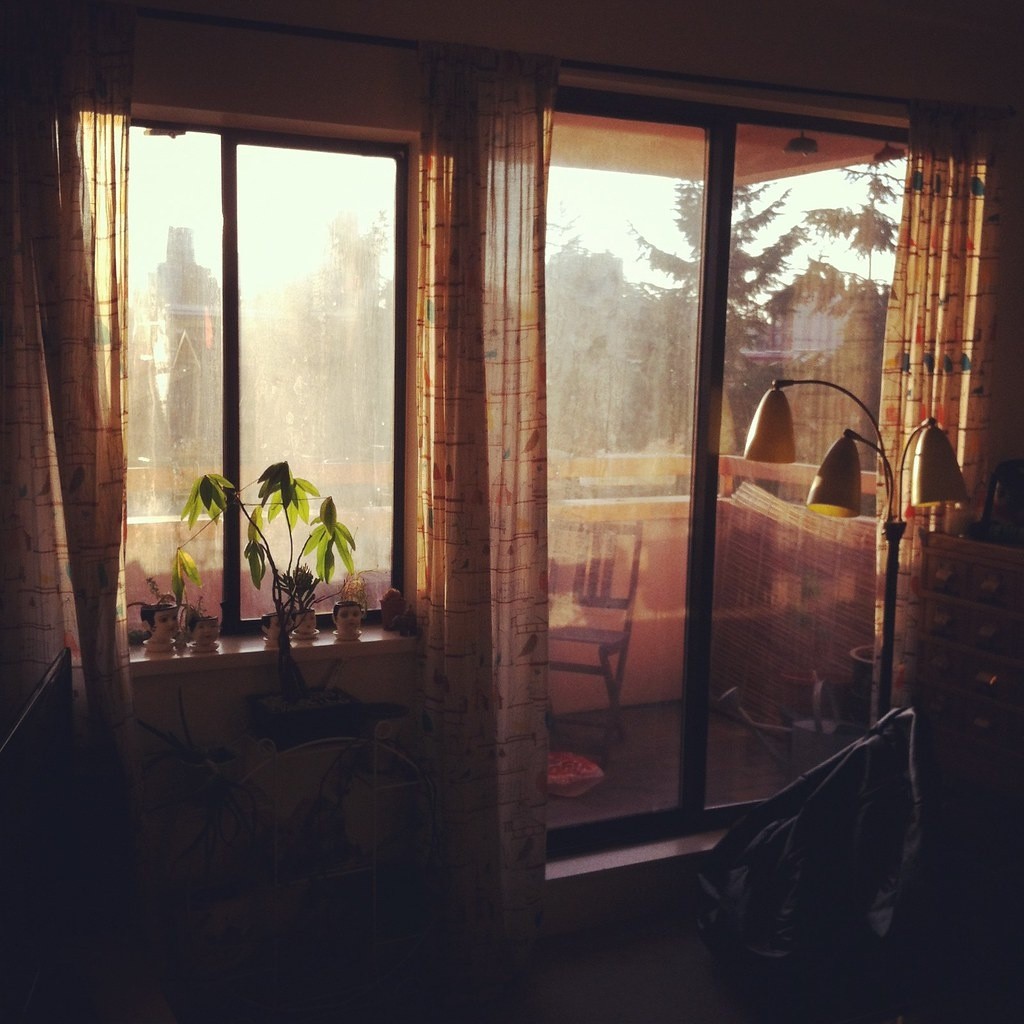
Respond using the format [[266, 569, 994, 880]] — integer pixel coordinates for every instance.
[[278, 567, 322, 640], [181, 597, 222, 653], [138, 579, 179, 653], [377, 588, 408, 631], [331, 568, 371, 641], [186, 461, 361, 755]]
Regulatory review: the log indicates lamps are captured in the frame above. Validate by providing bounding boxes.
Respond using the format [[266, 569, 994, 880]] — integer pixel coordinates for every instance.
[[743, 371, 967, 729]]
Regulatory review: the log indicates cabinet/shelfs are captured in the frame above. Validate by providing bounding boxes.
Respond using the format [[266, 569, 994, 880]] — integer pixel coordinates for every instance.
[[927, 528, 1024, 834]]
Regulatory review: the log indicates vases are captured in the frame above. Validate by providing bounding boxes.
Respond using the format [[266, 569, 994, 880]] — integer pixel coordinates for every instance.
[[842, 643, 876, 729], [364, 701, 409, 755], [261, 611, 294, 650]]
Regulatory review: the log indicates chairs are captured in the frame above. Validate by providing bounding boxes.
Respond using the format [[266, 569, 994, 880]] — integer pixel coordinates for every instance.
[[159, 736, 437, 1024], [547, 515, 643, 769]]
